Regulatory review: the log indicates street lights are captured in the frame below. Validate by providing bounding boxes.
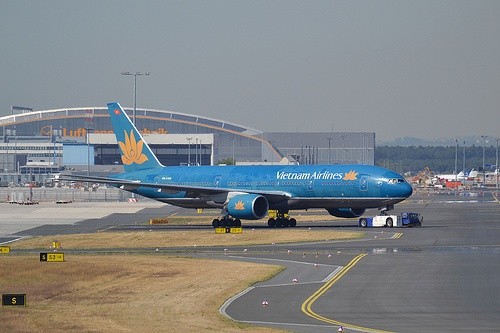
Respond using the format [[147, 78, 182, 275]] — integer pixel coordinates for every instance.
[[326, 136, 333, 165], [341, 134, 347, 162], [121, 71, 151, 126], [454, 135, 500, 198], [85, 127, 94, 187], [186, 136, 192, 166]]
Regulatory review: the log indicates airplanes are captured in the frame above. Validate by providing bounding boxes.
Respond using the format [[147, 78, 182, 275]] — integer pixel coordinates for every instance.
[[53, 102, 413, 228]]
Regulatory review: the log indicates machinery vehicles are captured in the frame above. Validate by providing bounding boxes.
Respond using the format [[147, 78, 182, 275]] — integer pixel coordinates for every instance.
[[358, 212, 424, 228]]
